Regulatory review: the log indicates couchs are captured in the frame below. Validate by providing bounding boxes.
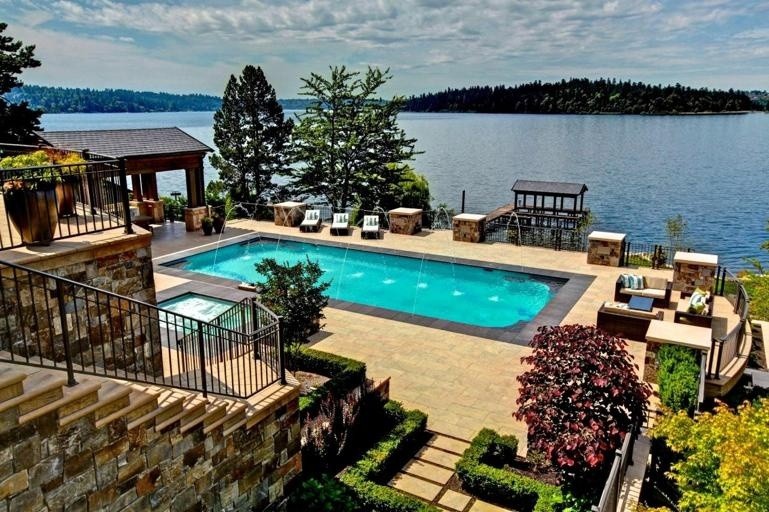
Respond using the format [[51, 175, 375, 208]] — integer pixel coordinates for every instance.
[[613, 271, 673, 307], [596, 301, 664, 336], [673, 282, 715, 328]]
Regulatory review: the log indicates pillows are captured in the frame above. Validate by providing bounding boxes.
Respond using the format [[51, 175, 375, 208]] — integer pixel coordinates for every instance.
[[688, 286, 710, 315], [621, 272, 667, 290]]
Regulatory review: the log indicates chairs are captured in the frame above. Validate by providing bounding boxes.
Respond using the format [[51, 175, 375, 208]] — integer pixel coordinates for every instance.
[[300, 208, 381, 239]]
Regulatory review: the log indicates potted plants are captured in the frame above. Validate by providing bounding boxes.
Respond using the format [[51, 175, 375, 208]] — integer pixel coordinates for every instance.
[[201, 215, 214, 234], [0, 145, 89, 248]]
